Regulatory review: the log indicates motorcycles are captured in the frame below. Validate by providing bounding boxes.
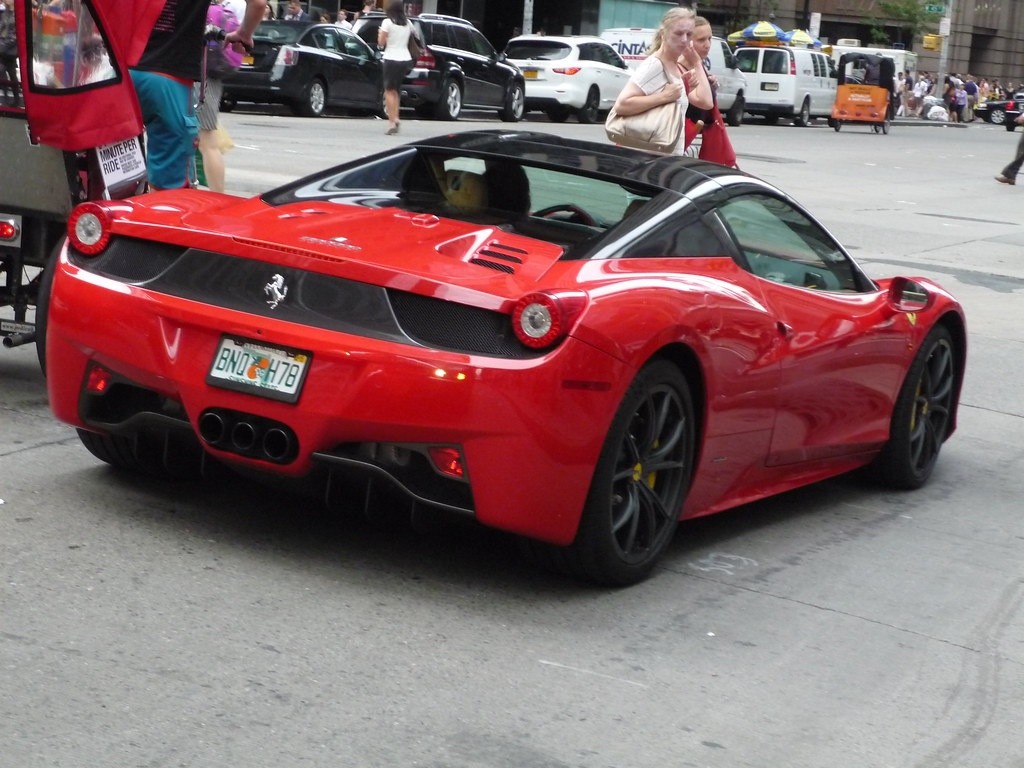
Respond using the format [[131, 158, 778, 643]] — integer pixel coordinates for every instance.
[[0, 0, 259, 375]]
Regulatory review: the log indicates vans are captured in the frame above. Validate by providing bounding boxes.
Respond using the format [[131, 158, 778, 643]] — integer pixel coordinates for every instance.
[[735, 41, 841, 129], [601, 29, 746, 127]]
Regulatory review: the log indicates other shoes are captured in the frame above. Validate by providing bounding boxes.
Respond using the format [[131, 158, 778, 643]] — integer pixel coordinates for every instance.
[[385, 119, 399, 135], [995, 175, 1015, 185], [10, 99, 21, 107]]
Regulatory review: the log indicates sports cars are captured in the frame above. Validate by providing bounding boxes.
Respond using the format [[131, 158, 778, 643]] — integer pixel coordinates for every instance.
[[46, 128, 965, 587]]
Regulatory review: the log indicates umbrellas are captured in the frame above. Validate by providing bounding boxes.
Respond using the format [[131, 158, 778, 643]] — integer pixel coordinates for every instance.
[[728, 21, 823, 50]]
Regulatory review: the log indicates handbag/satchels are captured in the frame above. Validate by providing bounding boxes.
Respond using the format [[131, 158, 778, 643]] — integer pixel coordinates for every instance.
[[406, 20, 421, 59], [699, 67, 737, 167], [675, 60, 698, 153], [604, 56, 683, 153]]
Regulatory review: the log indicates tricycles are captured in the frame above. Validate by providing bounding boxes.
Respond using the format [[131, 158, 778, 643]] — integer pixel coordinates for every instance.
[[831, 53, 894, 135]]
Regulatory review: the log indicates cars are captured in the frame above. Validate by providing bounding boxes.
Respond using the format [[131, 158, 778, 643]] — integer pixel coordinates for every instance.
[[218, 18, 393, 119], [506, 33, 629, 123], [349, 12, 525, 123], [973, 89, 1024, 126]]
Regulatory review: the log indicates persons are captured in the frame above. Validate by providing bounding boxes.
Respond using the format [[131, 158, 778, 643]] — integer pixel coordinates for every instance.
[[896, 70, 1024, 123], [0, 0, 421, 199], [616, 7, 719, 160], [994, 112, 1024, 184]]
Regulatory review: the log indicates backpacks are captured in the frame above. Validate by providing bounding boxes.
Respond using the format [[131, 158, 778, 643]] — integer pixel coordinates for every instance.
[[204, 5, 244, 81], [1, 9, 18, 58]]
